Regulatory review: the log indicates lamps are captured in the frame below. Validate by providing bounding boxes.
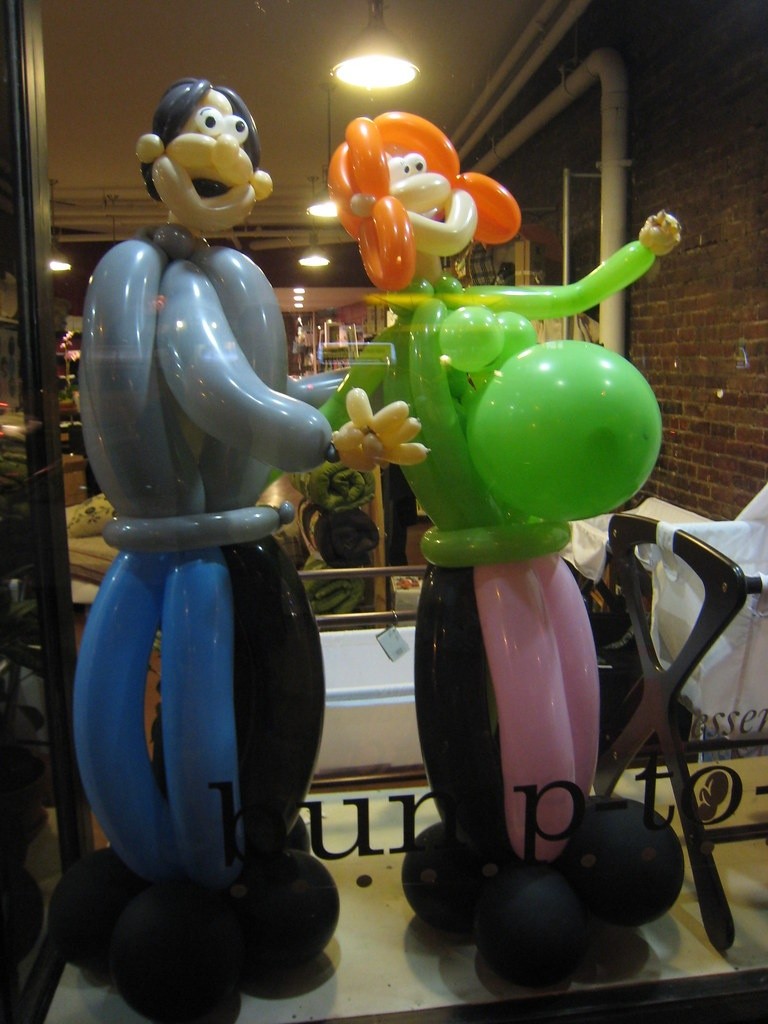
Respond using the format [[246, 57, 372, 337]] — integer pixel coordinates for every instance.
[[298, 175, 331, 266], [328, 0, 420, 91], [48, 179, 71, 271], [304, 83, 339, 218]]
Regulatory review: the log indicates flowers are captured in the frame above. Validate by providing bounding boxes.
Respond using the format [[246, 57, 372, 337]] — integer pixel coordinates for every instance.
[[60, 329, 81, 404]]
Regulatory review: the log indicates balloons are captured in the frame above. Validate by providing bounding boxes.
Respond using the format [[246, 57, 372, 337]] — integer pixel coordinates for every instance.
[[49, 78, 685, 1024]]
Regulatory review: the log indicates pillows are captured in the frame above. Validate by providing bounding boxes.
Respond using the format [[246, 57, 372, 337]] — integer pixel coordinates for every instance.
[[67, 493, 117, 538]]
